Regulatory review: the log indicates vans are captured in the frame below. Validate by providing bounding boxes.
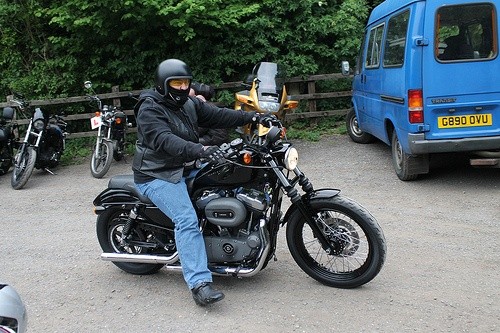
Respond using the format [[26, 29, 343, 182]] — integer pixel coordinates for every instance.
[[341, 0, 500, 182]]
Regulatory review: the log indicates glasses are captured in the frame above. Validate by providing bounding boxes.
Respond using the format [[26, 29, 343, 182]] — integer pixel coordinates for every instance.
[[169, 79, 190, 87]]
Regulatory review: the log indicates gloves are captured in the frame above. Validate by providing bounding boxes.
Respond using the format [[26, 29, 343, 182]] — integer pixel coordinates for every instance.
[[200, 145, 226, 164], [252, 112, 282, 128]]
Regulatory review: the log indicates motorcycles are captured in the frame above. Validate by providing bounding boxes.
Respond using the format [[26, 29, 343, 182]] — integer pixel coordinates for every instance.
[[83, 80, 134, 179], [0, 106, 21, 177], [231, 61, 298, 145], [93, 112, 387, 289], [9, 91, 69, 190]]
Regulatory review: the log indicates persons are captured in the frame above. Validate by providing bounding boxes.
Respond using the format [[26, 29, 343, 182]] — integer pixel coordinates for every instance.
[[132, 58, 278, 307]]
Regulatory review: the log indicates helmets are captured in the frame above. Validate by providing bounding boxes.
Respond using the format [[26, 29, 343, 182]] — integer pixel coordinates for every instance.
[[155, 59, 193, 106]]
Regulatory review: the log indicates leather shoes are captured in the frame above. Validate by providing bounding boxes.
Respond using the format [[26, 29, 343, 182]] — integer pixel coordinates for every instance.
[[192, 282, 225, 306]]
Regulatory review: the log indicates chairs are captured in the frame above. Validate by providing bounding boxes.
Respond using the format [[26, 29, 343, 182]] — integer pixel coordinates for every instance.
[[442, 35, 474, 58]]
